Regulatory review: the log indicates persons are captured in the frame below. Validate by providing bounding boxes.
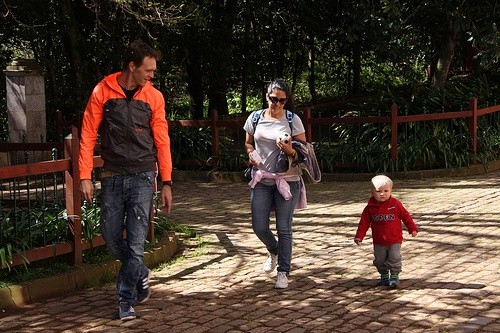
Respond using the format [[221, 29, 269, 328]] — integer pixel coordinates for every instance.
[[79, 40, 172, 320], [354, 174, 419, 287], [243, 79, 306, 289]]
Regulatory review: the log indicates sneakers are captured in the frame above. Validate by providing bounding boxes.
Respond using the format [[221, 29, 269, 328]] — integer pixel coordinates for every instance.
[[136, 269, 152, 303], [390, 274, 400, 287], [264, 253, 278, 273], [381, 274, 389, 284], [275, 271, 288, 288], [116, 301, 136, 321]]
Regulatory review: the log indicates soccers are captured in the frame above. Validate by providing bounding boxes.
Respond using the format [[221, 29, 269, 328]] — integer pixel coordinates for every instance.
[[275, 132, 292, 144]]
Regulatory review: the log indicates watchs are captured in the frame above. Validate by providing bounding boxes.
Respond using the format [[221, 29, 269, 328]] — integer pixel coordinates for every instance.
[[163, 180, 173, 186]]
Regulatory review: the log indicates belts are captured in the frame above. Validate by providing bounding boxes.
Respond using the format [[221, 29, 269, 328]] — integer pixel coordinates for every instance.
[[98, 170, 121, 178]]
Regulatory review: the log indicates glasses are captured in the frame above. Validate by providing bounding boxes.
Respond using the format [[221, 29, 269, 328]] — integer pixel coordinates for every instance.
[[267, 94, 288, 104]]
